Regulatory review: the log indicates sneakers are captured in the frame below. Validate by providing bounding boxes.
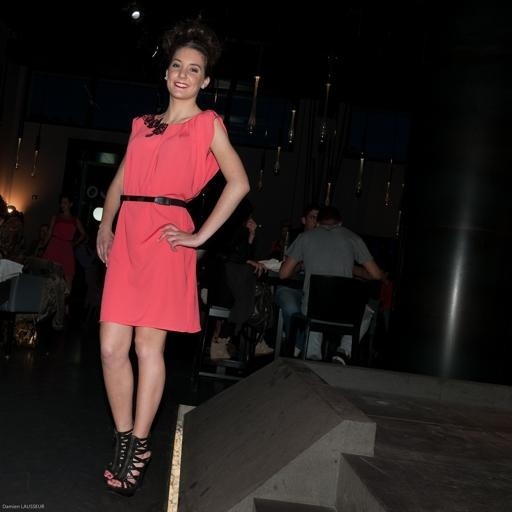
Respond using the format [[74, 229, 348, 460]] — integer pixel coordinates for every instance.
[[330, 346, 351, 367]]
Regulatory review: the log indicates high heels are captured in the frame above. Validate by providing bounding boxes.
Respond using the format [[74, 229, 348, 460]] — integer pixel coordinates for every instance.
[[104, 427, 154, 497]]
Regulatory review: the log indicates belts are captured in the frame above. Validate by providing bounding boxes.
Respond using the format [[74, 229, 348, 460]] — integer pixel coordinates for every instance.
[[122, 194, 186, 209]]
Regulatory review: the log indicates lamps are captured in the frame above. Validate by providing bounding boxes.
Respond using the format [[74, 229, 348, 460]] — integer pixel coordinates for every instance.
[[31, 124, 42, 177], [248, 75, 401, 209], [15, 120, 24, 168]]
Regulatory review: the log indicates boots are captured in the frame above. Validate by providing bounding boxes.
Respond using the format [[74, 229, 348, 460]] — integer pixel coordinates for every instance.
[[255, 333, 274, 355], [211, 336, 231, 359]]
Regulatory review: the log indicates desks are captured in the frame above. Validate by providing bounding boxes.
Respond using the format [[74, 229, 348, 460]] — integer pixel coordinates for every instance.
[[264, 277, 303, 359]]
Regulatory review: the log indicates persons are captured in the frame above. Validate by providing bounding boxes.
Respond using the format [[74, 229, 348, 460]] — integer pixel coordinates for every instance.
[[1, 193, 86, 284], [198, 201, 382, 366], [95, 28, 249, 497]]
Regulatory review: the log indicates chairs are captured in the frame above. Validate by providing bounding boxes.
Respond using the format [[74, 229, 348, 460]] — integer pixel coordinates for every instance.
[[186, 239, 269, 392], [287, 275, 369, 360]]
